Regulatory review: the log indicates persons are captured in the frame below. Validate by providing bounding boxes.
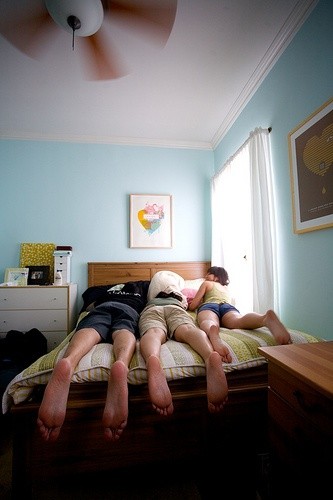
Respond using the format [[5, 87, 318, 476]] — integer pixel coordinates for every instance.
[[187, 265, 294, 363], [137, 285, 229, 416], [37, 279, 150, 441]]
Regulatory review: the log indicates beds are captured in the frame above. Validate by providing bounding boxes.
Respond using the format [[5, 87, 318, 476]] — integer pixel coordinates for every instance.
[[2, 261, 328, 500]]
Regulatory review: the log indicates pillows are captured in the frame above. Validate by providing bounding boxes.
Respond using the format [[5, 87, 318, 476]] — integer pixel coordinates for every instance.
[[180, 278, 206, 301]]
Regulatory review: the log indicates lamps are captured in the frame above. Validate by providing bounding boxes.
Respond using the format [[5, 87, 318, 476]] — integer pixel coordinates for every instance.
[[45, 0, 104, 51]]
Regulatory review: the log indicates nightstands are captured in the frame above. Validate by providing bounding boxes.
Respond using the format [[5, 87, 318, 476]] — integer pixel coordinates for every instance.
[[0, 283, 78, 353]]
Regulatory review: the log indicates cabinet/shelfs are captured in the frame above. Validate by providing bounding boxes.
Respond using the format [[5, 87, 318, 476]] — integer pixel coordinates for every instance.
[[257, 341, 333, 472]]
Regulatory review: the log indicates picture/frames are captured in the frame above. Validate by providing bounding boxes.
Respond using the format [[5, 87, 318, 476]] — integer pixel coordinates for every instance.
[[129, 194, 172, 249], [4, 268, 28, 286], [26, 266, 49, 285], [288, 97, 333, 234]]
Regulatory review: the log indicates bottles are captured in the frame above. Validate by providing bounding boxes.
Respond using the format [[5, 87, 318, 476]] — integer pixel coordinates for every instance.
[[20, 273, 27, 286], [55, 270, 62, 286]]
